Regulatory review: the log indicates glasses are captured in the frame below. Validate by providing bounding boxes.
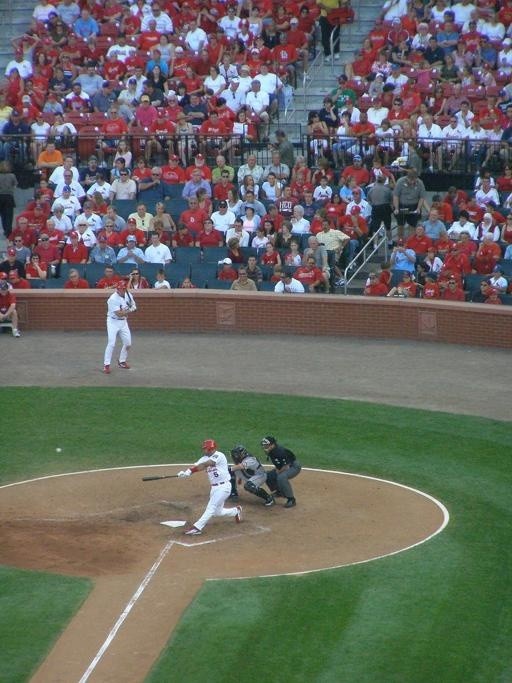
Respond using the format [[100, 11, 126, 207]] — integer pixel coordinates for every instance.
[[131, 272, 138, 274], [110, 111, 115, 113], [393, 102, 399, 105]]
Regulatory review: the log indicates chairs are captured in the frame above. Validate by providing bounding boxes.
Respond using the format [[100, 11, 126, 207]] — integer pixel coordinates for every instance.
[[0, 318, 12, 330]]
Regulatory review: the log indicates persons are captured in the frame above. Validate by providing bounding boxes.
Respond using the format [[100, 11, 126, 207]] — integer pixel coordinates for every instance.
[[227, 447, 275, 507], [261, 436, 301, 508], [103, 280, 137, 375], [176, 439, 242, 537], [1, 1, 512, 339]]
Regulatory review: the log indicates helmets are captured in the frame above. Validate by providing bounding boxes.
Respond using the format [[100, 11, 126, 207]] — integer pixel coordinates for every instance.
[[202, 439, 216, 449], [230, 446, 248, 463], [260, 436, 277, 453], [116, 280, 127, 289]]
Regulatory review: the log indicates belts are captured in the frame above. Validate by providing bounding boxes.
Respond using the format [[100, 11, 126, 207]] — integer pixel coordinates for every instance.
[[211, 481, 223, 485], [111, 316, 125, 320]]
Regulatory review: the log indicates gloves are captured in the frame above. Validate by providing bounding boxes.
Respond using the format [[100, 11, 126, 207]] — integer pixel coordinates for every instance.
[[177, 469, 191, 478]]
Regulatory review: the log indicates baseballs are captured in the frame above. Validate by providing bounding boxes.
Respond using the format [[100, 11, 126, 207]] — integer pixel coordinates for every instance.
[[56, 446, 62, 453]]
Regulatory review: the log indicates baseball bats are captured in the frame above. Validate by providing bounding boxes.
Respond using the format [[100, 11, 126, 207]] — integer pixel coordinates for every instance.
[[142, 474, 179, 481]]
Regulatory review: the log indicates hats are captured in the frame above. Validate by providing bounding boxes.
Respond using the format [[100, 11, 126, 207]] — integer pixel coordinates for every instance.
[[392, 17, 401, 23], [397, 236, 503, 283], [176, 201, 360, 233], [484, 200, 496, 208], [128, 77, 189, 119], [323, 61, 400, 103], [230, 47, 259, 83], [0, 282, 7, 289], [1, 200, 172, 278], [14, 48, 22, 53], [9, 92, 57, 117], [102, 79, 113, 88], [289, 16, 298, 23], [221, 258, 293, 278], [30, 154, 367, 200], [483, 37, 511, 96]]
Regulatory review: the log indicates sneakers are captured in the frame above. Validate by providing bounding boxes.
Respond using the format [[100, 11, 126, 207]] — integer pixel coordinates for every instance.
[[11, 328, 21, 338], [285, 499, 296, 507], [272, 490, 280, 496], [103, 365, 110, 375], [231, 492, 239, 497], [234, 506, 242, 523], [265, 499, 275, 506], [118, 362, 128, 369], [185, 527, 201, 536]]
